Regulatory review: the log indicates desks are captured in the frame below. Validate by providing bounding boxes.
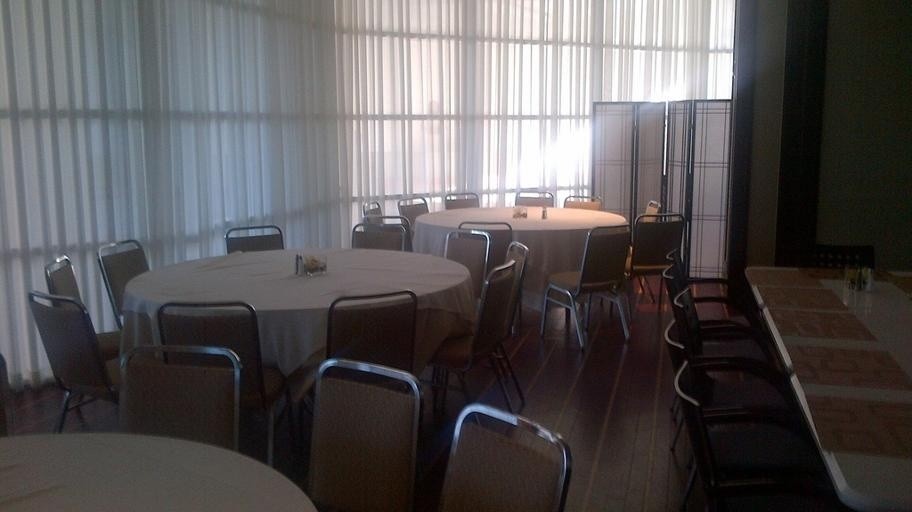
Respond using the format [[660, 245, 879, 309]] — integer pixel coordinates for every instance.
[[738, 264, 911, 510], [1, 434, 315, 511]]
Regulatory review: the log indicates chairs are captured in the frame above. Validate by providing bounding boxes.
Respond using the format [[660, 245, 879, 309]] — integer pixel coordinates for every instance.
[[438, 404, 574, 509], [29, 215, 530, 469], [309, 358, 423, 510], [663, 248, 839, 509], [363, 188, 692, 352], [117, 343, 242, 453]]
